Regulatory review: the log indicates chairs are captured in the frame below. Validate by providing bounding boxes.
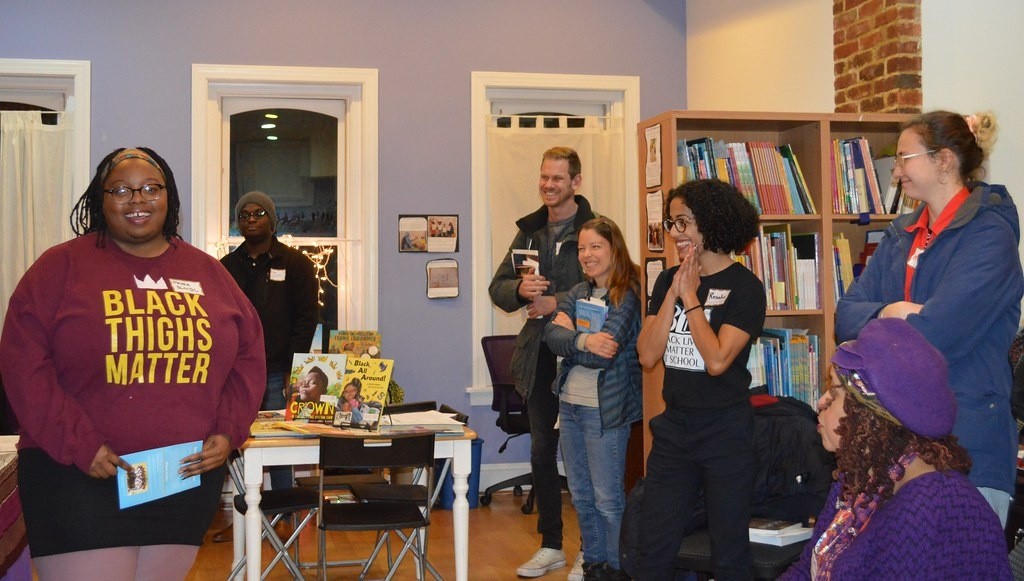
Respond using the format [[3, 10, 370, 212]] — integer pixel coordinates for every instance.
[[224, 401, 471, 581], [479, 334, 573, 515]]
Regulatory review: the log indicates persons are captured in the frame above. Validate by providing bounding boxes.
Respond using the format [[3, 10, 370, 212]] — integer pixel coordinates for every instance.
[[777, 318, 1013, 581], [488, 147, 603, 581], [213, 192, 320, 545], [637, 179, 767, 581], [288, 365, 334, 424], [835, 111, 1024, 528], [0, 146, 267, 581], [544, 217, 644, 581]]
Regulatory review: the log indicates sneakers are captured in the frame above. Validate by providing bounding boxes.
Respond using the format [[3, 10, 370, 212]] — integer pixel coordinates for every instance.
[[567, 550, 588, 581], [516, 547, 566, 577]]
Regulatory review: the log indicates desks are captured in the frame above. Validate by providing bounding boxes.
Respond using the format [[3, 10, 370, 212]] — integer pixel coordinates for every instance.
[[232, 413, 476, 581]]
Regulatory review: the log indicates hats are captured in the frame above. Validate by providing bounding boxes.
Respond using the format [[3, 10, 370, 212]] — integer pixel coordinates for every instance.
[[234, 190, 278, 233], [830, 318, 958, 438]]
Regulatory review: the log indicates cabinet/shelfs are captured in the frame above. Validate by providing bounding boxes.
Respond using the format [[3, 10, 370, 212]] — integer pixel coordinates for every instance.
[[637, 109, 921, 476]]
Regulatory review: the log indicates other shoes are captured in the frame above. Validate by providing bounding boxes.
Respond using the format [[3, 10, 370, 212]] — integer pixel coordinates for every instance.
[[581, 561, 630, 581]]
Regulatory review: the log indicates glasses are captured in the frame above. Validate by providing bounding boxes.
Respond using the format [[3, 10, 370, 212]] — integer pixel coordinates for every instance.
[[662, 217, 694, 233], [104, 181, 168, 204], [238, 209, 266, 221], [892, 149, 938, 167]]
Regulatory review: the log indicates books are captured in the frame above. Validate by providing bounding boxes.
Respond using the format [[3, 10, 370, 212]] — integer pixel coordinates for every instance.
[[285, 353, 395, 433], [677, 137, 817, 215], [511, 249, 543, 318], [747, 328, 822, 412], [830, 137, 922, 214], [575, 298, 609, 334], [729, 224, 821, 311], [832, 231, 854, 308]]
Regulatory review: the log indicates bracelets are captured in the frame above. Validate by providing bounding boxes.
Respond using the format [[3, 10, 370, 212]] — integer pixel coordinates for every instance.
[[684, 305, 702, 314]]
